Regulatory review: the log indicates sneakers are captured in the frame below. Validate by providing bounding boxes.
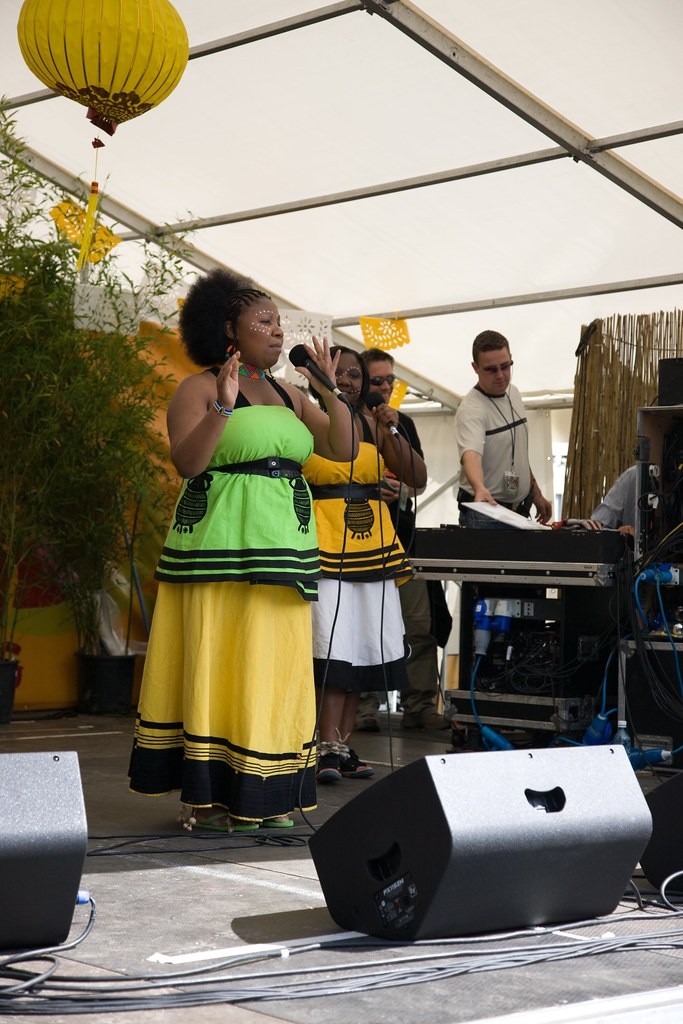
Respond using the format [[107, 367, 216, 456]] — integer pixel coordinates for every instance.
[[335, 727, 374, 780], [400, 706, 452, 729], [317, 741, 342, 783], [354, 702, 380, 731]]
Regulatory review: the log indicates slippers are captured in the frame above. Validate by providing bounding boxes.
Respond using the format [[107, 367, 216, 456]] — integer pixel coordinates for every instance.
[[191, 809, 259, 831], [259, 817, 294, 828]]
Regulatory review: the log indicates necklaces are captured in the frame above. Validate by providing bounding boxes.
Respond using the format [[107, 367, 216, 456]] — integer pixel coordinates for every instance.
[[216, 357, 266, 379]]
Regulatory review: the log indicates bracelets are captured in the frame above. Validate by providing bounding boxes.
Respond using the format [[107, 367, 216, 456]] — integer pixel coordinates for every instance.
[[211, 400, 233, 417]]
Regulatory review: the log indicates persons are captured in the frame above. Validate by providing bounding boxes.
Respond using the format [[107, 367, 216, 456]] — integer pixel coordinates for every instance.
[[127, 267, 360, 831], [454, 331, 552, 673], [590, 465, 636, 538], [301, 344, 451, 783]]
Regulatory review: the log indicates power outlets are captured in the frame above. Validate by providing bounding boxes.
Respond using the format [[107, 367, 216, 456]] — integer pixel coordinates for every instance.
[[577, 634, 600, 664], [651, 566, 680, 586], [485, 598, 521, 619]]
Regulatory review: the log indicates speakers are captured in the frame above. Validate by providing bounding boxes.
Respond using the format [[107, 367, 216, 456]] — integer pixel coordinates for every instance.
[[0, 752, 88, 958], [306, 743, 655, 944]]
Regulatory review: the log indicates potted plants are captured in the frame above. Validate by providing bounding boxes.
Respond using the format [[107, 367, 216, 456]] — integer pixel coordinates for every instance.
[[0, 94, 206, 726]]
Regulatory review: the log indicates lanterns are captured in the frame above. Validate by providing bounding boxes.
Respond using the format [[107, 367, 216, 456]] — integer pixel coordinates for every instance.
[[17, 0, 190, 272]]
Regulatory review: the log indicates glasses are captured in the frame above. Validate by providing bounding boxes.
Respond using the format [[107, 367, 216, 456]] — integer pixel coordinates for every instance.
[[482, 358, 512, 373], [369, 374, 394, 386]]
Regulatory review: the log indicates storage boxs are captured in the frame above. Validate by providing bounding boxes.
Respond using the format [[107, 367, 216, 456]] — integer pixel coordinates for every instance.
[[443, 688, 593, 732], [618, 638, 683, 775]]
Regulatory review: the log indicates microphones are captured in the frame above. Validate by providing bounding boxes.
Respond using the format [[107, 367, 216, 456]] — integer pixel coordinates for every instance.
[[289, 344, 347, 406], [365, 392, 400, 440]]
